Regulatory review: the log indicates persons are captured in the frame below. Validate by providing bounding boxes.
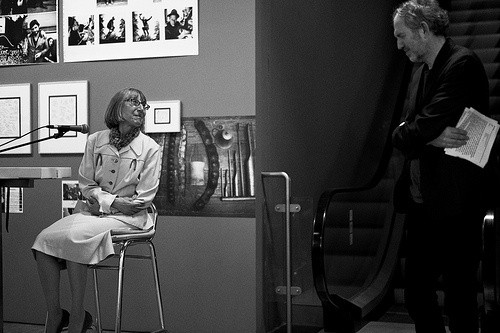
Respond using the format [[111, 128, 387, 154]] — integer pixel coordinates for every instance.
[[165, 8, 191, 39], [32, 88, 162, 333], [69, 17, 94, 45], [99, 14, 125, 39], [27, 19, 56, 63], [392, 0, 489, 333], [140, 14, 152, 36]]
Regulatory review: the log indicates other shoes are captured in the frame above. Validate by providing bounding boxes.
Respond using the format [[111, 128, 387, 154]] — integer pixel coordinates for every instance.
[[81, 310, 92, 333], [45, 309, 70, 333]]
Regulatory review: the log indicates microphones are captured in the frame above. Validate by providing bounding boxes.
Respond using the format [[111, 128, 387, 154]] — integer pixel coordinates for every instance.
[[48, 124, 89, 134]]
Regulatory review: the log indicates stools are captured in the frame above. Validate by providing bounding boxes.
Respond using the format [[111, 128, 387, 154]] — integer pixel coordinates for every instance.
[[44, 200, 165, 333]]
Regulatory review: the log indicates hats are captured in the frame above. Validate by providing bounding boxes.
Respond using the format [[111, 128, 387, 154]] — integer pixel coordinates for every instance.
[[167, 9, 179, 19], [68, 16, 75, 32]]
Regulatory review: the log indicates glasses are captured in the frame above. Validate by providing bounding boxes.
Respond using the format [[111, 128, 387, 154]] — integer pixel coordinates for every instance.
[[123, 98, 150, 110]]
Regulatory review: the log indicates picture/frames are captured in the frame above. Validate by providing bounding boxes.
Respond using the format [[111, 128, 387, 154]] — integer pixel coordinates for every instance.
[[144, 99, 182, 133], [37, 80, 90, 155], [0, 82, 32, 155]]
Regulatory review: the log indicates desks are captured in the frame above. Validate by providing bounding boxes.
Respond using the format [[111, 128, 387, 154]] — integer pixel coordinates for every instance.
[[0, 166, 72, 333]]
